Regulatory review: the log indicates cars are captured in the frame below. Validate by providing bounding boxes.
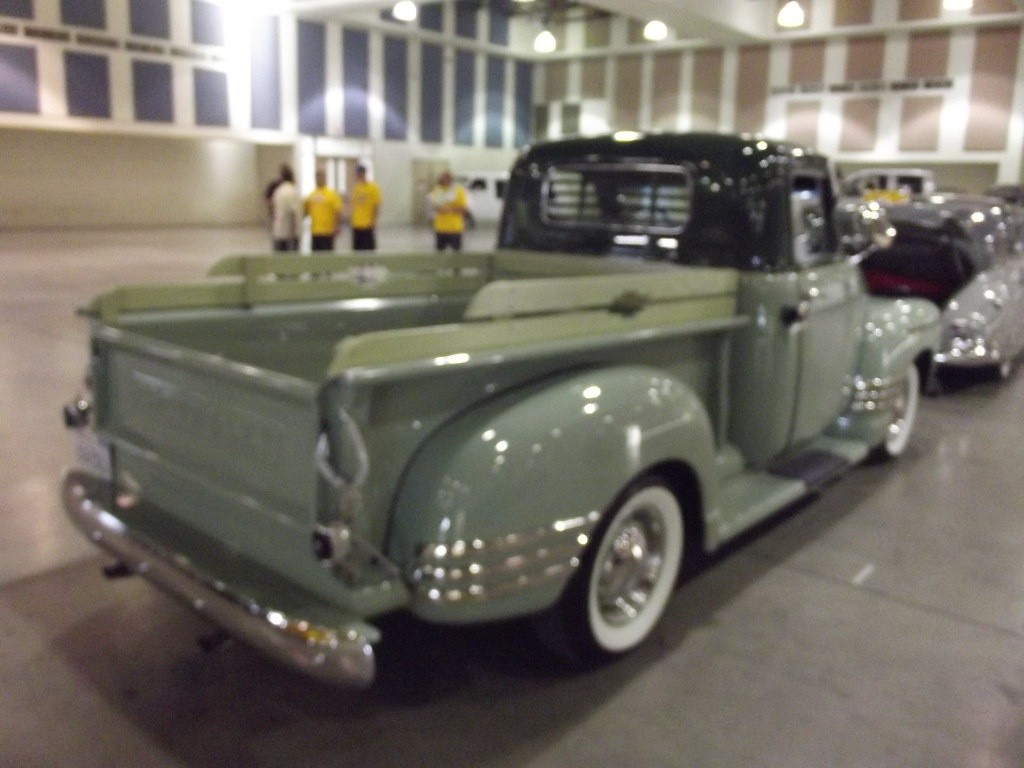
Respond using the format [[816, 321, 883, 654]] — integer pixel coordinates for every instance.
[[796, 165, 1023, 393]]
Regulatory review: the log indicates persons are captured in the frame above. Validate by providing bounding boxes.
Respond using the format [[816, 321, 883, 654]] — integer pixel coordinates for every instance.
[[302, 170, 345, 250], [266, 164, 303, 252], [347, 165, 381, 250], [430, 170, 467, 250]]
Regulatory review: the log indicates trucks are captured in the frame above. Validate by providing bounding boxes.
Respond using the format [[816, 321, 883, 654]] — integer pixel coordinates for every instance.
[[62, 130, 946, 702]]
